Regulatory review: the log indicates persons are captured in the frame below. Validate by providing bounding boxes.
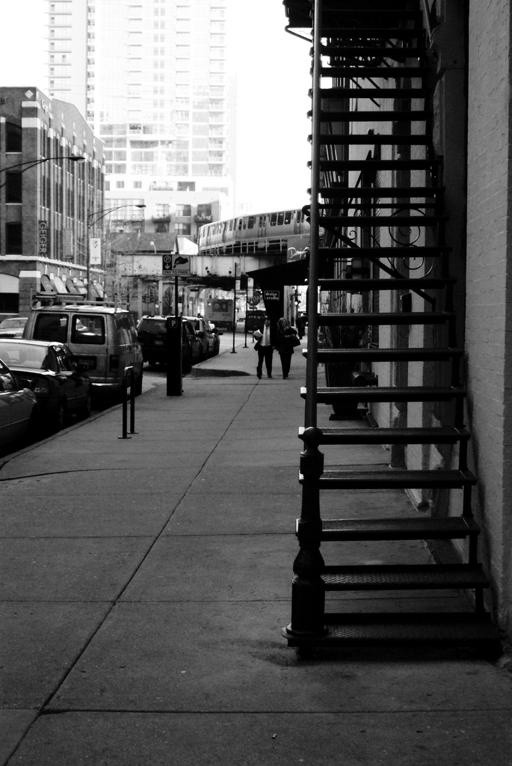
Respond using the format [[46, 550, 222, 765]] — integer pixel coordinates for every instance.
[[254, 312, 309, 378]]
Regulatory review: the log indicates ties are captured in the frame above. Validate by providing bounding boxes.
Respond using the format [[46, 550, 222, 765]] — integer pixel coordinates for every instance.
[[264, 326, 268, 345]]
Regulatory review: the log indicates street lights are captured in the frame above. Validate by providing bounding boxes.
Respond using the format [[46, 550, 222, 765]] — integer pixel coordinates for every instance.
[[2, 154, 88, 189], [85, 202, 147, 301]]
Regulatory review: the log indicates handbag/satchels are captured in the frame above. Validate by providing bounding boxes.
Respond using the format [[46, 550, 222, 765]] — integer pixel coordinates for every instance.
[[293, 337, 300, 346]]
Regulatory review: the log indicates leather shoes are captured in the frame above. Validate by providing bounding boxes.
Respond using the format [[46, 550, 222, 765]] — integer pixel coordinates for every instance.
[[256, 373, 272, 379]]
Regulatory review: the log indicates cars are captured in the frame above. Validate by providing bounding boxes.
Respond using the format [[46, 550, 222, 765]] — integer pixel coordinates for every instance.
[[0, 338, 97, 431], [184, 316, 219, 361], [136, 314, 199, 375]]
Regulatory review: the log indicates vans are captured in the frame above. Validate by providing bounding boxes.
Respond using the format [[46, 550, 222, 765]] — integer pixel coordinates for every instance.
[[22, 303, 147, 389]]
